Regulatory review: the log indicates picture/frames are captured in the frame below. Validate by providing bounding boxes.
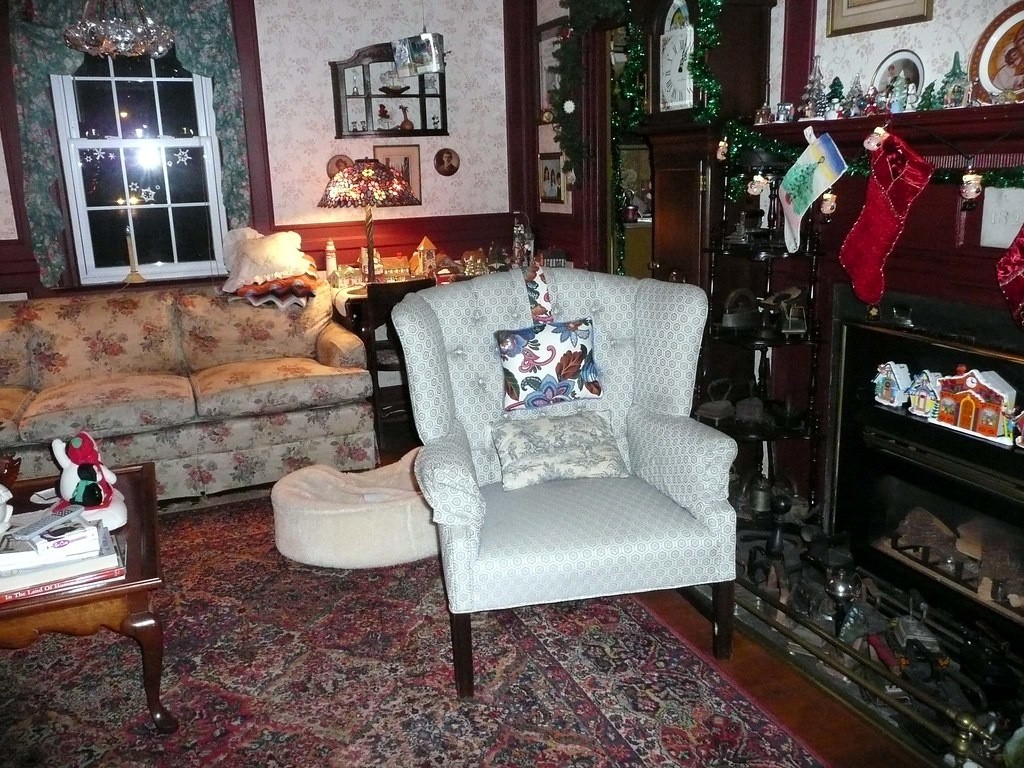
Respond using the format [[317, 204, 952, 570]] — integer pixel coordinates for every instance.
[[825, 0, 932, 39], [373, 144, 422, 206], [536, 152, 566, 204], [537, 13, 570, 125]]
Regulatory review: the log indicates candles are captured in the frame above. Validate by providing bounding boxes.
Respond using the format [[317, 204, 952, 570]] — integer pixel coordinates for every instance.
[[126, 233, 135, 271]]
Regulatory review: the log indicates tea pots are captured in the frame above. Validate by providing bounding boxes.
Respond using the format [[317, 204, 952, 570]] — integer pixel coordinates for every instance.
[[824, 568, 858, 605]]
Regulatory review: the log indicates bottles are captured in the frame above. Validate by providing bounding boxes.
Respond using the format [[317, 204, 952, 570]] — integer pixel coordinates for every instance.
[[325, 240, 337, 279]]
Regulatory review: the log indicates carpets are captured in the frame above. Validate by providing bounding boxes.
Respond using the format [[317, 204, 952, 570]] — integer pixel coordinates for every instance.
[[0, 469, 836, 768]]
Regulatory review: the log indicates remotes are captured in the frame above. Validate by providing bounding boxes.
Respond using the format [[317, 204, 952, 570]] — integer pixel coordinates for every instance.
[[11, 504, 85, 540]]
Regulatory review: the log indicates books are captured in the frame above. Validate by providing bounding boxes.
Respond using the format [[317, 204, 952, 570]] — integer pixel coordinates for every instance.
[[0, 519, 127, 603]]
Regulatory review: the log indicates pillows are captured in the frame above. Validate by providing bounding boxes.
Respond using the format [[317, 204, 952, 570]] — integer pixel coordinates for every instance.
[[497, 318, 601, 409], [490, 409, 630, 491]]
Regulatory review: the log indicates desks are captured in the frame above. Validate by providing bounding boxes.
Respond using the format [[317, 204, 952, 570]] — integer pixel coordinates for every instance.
[[326, 280, 408, 422]]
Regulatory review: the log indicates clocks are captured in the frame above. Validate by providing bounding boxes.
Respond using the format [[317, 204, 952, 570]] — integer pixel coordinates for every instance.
[[639, 0, 777, 295]]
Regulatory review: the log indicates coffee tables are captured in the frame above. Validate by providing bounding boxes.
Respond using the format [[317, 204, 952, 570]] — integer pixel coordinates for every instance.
[[1, 462, 181, 734]]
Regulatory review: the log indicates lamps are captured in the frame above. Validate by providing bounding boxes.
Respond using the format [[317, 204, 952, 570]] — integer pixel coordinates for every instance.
[[319, 157, 421, 452]]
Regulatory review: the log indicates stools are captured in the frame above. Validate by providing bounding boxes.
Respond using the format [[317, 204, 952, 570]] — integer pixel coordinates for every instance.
[[273, 441, 433, 569]]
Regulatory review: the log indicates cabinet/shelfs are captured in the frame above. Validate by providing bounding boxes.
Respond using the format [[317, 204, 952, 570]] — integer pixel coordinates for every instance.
[[749, 135, 1024, 768], [622, 227, 654, 277], [328, 42, 449, 140]]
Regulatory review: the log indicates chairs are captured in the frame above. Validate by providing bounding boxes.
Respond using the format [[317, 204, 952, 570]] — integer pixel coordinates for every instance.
[[389, 264, 738, 698], [367, 276, 437, 449]]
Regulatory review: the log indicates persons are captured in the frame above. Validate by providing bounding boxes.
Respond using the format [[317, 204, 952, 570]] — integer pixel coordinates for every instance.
[[541, 166, 561, 197], [438, 151, 457, 176]]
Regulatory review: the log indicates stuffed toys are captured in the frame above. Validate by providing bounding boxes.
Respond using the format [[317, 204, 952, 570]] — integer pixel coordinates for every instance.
[[52, 431, 117, 506]]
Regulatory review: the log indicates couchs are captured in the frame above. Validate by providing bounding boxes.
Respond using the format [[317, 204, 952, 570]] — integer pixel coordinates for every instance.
[[0, 284, 379, 513]]
